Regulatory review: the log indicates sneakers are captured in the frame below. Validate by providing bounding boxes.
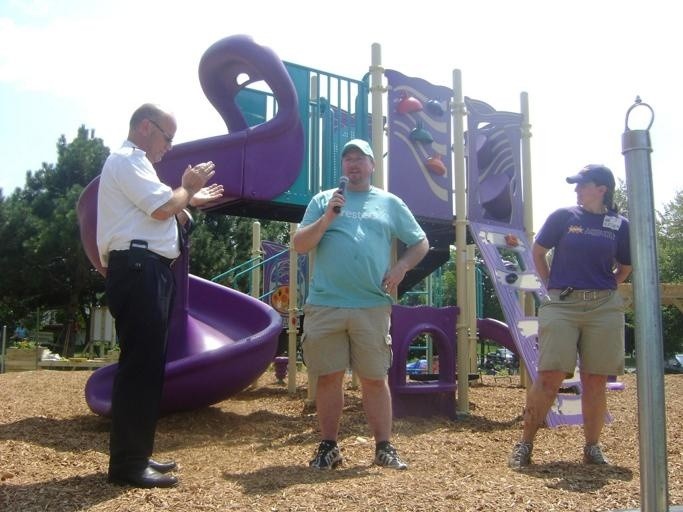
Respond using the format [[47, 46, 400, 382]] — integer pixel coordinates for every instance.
[[309, 441, 342, 471], [584, 444, 622, 474], [510, 441, 533, 468], [375, 441, 408, 470]]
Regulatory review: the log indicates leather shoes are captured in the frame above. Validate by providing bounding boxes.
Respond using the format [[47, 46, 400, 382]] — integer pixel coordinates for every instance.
[[109, 458, 178, 488]]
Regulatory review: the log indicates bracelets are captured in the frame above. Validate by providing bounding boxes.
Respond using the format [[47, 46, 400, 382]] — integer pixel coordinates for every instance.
[[185, 203, 196, 212]]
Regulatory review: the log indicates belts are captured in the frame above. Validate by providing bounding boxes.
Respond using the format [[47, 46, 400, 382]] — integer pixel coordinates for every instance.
[[108, 250, 176, 271]]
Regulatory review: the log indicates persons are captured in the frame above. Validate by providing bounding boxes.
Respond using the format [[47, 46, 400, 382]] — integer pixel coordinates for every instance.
[[8, 319, 29, 342], [94, 100, 228, 489], [506, 157, 629, 472], [285, 133, 428, 471]]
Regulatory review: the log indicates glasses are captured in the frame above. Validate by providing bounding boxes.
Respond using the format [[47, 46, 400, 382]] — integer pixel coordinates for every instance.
[[149, 119, 172, 144]]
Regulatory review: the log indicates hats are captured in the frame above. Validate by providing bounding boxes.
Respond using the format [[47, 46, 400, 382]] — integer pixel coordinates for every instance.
[[566, 164, 614, 185], [341, 138, 372, 157]]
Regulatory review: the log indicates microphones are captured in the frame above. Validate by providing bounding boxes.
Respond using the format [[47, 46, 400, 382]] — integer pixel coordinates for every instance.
[[334, 175, 349, 213]]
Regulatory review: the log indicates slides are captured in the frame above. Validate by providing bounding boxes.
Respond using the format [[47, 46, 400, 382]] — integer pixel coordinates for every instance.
[[77, 34, 306, 418]]
